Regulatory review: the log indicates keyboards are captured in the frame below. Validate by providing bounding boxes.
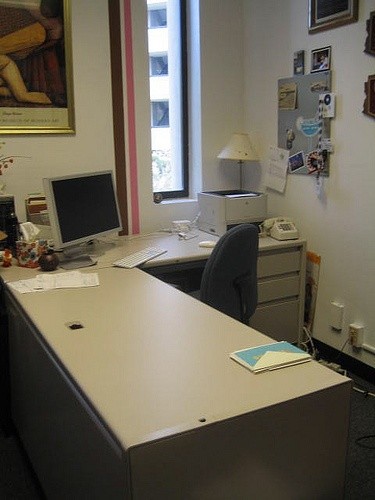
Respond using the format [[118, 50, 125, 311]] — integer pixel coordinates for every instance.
[[112, 244, 168, 270]]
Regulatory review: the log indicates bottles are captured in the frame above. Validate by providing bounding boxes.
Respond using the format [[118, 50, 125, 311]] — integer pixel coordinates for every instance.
[[38, 254, 59, 271]]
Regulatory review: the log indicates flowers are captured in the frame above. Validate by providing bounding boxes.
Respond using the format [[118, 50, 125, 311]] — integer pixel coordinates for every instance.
[[0, 141, 34, 176]]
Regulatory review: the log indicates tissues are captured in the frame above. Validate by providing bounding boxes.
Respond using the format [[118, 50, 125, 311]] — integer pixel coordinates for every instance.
[[15, 221, 42, 269]]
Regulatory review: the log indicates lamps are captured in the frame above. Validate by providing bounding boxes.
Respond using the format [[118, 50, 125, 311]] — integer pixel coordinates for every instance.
[[216, 132, 259, 192]]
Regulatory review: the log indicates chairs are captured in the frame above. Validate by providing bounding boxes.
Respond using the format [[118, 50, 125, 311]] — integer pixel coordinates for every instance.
[[187, 224, 259, 327]]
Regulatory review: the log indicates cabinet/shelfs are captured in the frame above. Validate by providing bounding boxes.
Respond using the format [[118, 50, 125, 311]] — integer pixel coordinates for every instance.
[[0, 230, 353, 499]]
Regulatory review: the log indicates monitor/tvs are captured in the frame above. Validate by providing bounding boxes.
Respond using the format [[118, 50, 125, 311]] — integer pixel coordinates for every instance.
[[42, 169, 124, 258]]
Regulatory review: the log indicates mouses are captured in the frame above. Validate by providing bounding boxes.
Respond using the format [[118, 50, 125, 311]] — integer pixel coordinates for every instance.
[[198, 241, 217, 248]]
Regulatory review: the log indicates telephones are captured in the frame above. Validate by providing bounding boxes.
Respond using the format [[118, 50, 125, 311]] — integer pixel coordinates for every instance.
[[264, 217, 299, 240]]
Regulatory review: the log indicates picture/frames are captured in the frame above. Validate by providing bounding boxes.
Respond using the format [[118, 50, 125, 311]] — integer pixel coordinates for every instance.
[[363, 11, 375, 55], [310, 46, 331, 73], [308, 0, 359, 35], [0, 0, 77, 134], [362, 74, 375, 118]]
